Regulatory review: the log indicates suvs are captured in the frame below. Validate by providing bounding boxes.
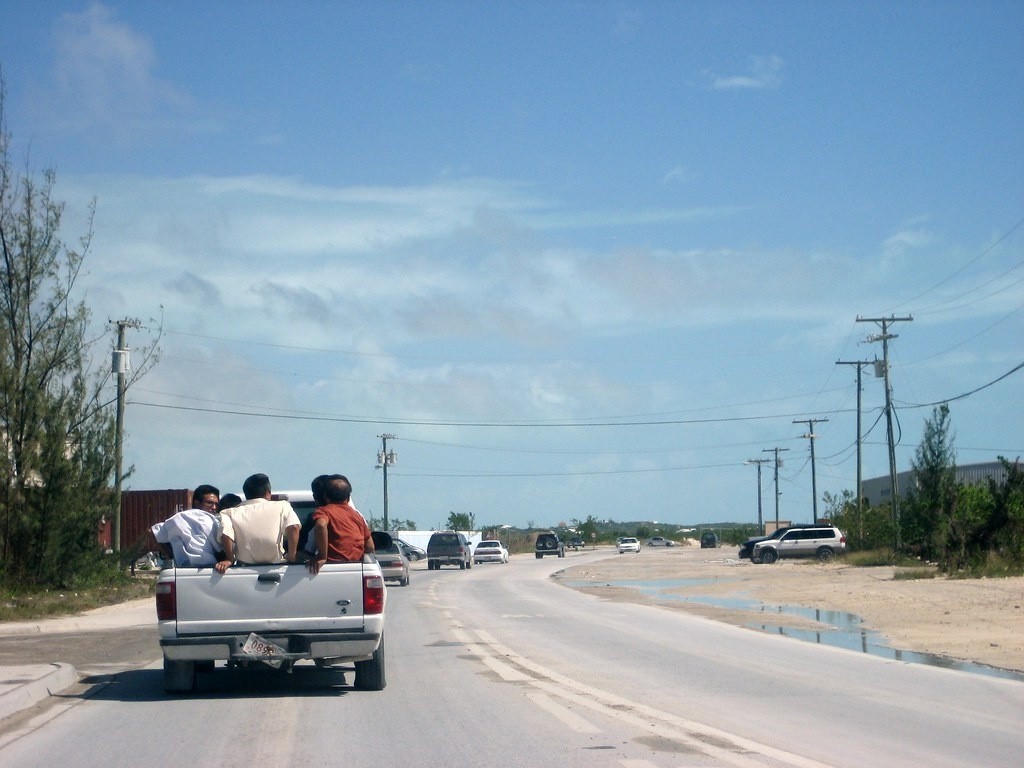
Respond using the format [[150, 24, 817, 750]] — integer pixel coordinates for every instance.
[[427, 532, 472, 570], [738, 524, 836, 562], [535, 534, 565, 558]]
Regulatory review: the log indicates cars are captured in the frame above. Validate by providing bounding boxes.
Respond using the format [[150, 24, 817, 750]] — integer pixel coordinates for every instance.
[[566, 537, 585, 548], [751, 527, 845, 564], [648, 537, 674, 546], [392, 538, 426, 561], [701, 532, 721, 548], [616, 537, 624, 548], [375, 541, 409, 586], [474, 540, 509, 564], [618, 537, 641, 554]]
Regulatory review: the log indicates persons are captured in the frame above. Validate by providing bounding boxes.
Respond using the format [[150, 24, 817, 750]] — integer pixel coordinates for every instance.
[[148, 485, 242, 568], [283, 474, 374, 574], [215, 474, 302, 574]]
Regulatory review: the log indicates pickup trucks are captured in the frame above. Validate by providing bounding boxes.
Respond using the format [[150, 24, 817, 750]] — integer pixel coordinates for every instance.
[[155, 490, 393, 690]]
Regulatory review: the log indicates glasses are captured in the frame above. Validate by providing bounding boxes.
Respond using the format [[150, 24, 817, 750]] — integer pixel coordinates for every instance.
[[202, 500, 224, 509]]
